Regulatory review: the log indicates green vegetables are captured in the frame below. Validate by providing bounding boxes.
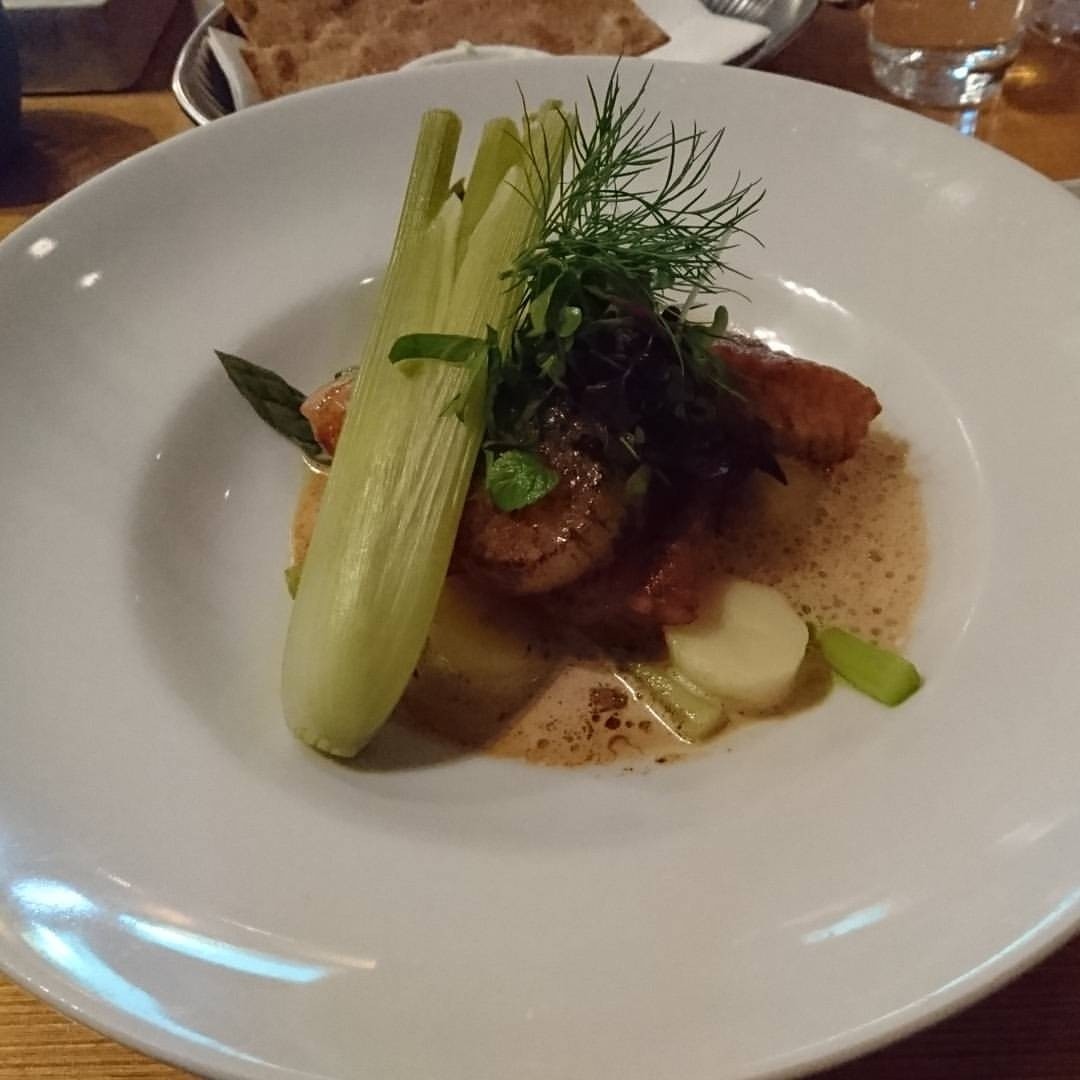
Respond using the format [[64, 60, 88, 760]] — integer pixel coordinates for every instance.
[[216, 42, 919, 757]]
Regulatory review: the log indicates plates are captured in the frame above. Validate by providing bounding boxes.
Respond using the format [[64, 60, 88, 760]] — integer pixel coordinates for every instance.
[[1, 57, 1080, 1078]]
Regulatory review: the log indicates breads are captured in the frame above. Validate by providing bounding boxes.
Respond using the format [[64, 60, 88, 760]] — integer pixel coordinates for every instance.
[[222, 0, 669, 97]]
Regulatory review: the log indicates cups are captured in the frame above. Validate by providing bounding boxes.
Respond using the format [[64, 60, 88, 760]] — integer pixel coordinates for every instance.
[[1019, 1, 1080, 49], [862, 0, 1034, 110]]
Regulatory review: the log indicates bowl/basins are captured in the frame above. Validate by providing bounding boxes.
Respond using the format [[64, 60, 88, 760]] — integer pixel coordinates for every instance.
[[167, 1, 822, 125]]
